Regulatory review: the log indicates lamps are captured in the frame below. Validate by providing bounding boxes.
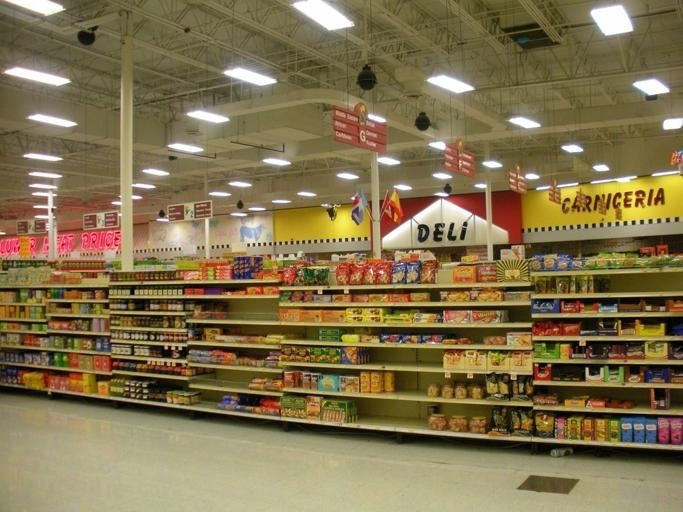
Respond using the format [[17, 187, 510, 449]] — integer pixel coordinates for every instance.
[[414, 112, 432, 131], [320, 201, 341, 220], [443, 183, 452, 194], [237, 200, 243, 209], [354, 64, 378, 91], [158, 209, 165, 218], [77, 26, 99, 46]]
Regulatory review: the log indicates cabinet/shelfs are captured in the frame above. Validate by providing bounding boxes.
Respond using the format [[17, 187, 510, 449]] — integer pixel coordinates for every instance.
[[0, 284, 110, 400], [531, 268, 683, 450], [278, 282, 531, 442], [108, 279, 278, 421]]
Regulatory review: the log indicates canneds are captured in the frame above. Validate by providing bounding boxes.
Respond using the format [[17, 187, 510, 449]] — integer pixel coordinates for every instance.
[[109, 285, 183, 295], [111, 344, 164, 357], [112, 359, 209, 377], [109, 299, 199, 312], [109, 271, 177, 282], [110, 314, 199, 328], [124, 380, 202, 406], [110, 329, 194, 342]]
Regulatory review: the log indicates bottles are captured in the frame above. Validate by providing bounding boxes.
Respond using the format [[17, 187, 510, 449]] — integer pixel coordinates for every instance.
[[110, 378, 124, 396]]
